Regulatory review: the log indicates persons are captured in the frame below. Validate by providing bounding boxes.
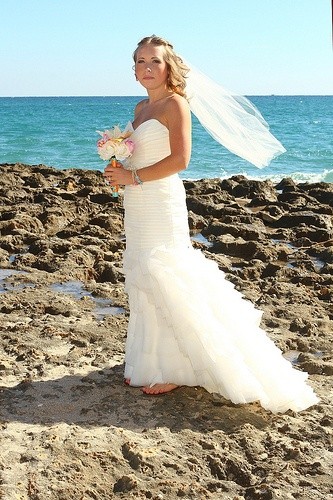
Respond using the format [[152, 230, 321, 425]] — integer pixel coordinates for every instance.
[[102, 34, 320, 414]]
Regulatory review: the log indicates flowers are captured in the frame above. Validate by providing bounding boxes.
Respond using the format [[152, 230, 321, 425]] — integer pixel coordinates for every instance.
[[93, 120, 136, 197]]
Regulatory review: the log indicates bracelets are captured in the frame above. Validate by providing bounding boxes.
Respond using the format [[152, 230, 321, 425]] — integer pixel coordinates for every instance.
[[131, 169, 136, 185], [134, 169, 143, 185]]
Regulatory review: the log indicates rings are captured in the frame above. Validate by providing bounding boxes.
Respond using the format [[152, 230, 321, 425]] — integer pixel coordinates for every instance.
[[108, 177, 112, 182]]
